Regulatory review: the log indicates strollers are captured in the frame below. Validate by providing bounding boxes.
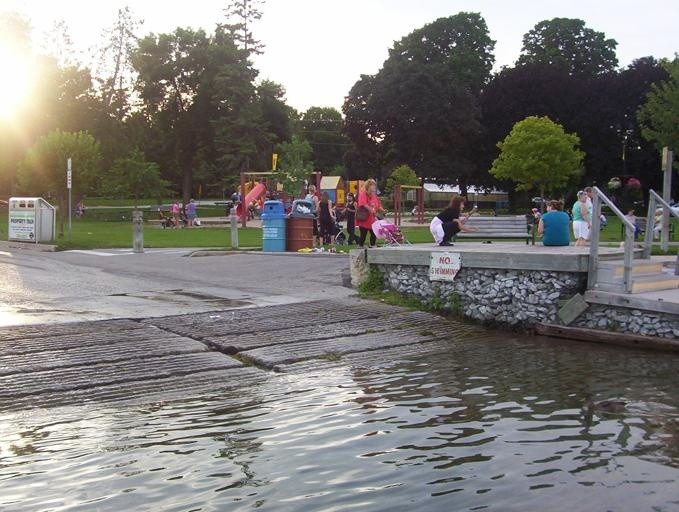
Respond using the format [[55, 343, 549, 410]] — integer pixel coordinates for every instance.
[[325, 224, 346, 246], [369, 209, 414, 247], [158, 208, 175, 229]]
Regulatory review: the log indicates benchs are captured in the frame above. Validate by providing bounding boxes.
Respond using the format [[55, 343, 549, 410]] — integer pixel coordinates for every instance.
[[622, 223, 673, 239], [453, 215, 536, 246]]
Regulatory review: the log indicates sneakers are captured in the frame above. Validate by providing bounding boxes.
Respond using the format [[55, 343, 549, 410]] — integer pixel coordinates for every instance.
[[356, 245, 365, 249], [369, 244, 378, 248], [440, 241, 453, 246]]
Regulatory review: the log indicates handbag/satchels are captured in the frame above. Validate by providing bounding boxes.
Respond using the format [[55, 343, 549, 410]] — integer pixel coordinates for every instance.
[[356, 207, 368, 221]]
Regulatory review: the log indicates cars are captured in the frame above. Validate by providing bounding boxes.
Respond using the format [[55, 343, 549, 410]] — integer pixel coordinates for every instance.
[[656, 203, 679, 218], [531, 196, 549, 208]]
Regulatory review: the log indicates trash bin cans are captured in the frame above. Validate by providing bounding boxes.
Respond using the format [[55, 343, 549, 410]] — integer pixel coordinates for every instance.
[[261, 200, 314, 252]]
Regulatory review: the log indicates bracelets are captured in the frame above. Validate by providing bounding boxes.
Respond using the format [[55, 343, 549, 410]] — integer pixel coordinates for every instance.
[[468, 211, 471, 215]]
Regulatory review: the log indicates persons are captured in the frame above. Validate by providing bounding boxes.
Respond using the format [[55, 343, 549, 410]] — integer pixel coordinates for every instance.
[[353, 178, 389, 248], [531, 185, 665, 246], [316, 192, 336, 246], [340, 192, 361, 245], [430, 194, 479, 246], [172, 200, 180, 229], [74, 200, 85, 216], [229, 184, 358, 224], [185, 198, 196, 227], [304, 184, 319, 240]]
